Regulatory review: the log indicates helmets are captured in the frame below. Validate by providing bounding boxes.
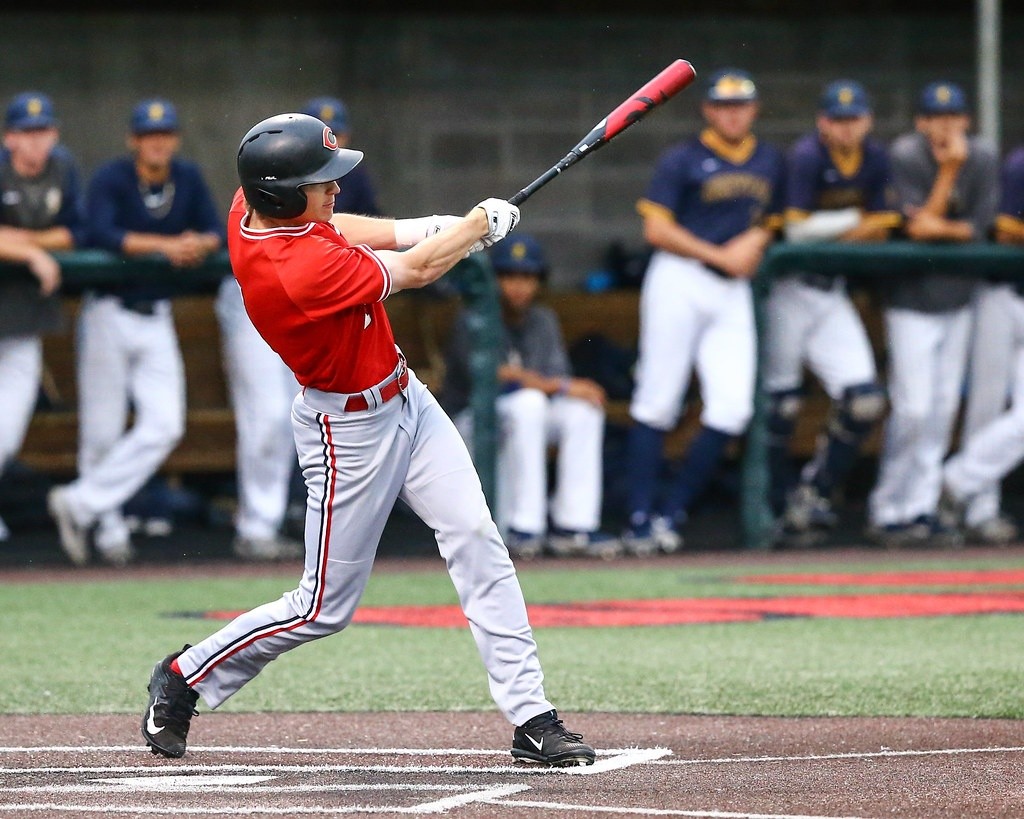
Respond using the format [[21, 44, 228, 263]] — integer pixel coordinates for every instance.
[[236, 113, 364, 220]]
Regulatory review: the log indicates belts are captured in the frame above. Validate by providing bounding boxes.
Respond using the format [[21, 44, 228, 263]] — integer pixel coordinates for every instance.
[[303, 354, 409, 412]]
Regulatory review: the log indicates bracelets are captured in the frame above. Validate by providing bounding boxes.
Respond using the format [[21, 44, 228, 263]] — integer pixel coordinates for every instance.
[[558, 376, 570, 396], [23, 245, 39, 266]]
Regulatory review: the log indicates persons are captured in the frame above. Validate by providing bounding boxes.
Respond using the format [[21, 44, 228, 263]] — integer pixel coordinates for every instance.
[[623, 74, 1024, 557], [212, 99, 379, 563], [140, 113, 596, 769], [438, 229, 609, 560], [0, 93, 226, 566]]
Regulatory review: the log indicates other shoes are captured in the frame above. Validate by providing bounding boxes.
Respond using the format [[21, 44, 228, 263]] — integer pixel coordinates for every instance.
[[786, 487, 815, 530], [870, 515, 966, 550], [232, 535, 304, 559], [966, 520, 1012, 547], [939, 491, 965, 528], [622, 514, 683, 554]]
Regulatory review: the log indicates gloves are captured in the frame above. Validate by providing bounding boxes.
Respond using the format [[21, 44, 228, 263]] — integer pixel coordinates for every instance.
[[476, 197, 521, 243], [426, 214, 493, 259]]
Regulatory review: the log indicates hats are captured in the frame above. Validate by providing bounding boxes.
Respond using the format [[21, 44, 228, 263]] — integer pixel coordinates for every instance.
[[818, 79, 871, 116], [494, 230, 544, 272], [303, 97, 349, 134], [704, 68, 758, 101], [130, 101, 182, 134], [920, 83, 966, 114], [6, 91, 59, 130]]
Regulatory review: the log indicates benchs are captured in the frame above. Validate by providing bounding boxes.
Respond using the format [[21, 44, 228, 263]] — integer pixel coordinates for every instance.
[[27, 293, 888, 495]]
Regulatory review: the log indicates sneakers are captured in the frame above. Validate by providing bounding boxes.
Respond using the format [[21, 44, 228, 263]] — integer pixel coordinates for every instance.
[[48, 486, 87, 566], [510, 709, 596, 767], [141, 644, 200, 758], [498, 526, 541, 558], [104, 545, 129, 565], [546, 525, 624, 559]]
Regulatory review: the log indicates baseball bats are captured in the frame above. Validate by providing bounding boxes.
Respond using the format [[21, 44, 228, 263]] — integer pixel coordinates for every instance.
[[507, 56, 698, 207]]
